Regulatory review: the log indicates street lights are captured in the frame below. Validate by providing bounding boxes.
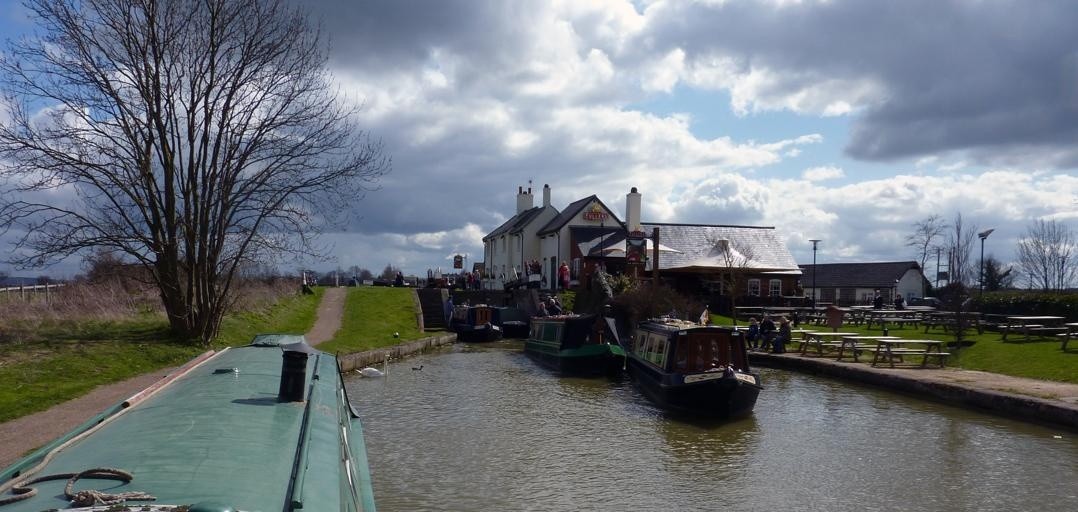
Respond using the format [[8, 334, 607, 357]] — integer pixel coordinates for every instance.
[[809, 239, 822, 322], [978, 229, 995, 296]]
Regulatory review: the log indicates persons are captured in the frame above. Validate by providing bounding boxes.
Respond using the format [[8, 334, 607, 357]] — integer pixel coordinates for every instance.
[[523, 260, 530, 277], [530, 257, 540, 275], [769, 316, 792, 355], [745, 317, 759, 349], [446, 269, 481, 290], [558, 260, 571, 292], [752, 312, 776, 352], [893, 295, 905, 312], [443, 295, 456, 332], [872, 290, 883, 310], [538, 295, 562, 342]]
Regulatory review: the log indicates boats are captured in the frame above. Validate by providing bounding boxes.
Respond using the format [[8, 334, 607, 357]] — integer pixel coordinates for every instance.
[[0, 337, 377, 511], [524, 303, 764, 415], [451, 304, 508, 342]]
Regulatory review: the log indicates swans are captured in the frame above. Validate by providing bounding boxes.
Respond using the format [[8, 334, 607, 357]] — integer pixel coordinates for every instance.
[[412, 365, 424, 370], [355, 354, 393, 378]]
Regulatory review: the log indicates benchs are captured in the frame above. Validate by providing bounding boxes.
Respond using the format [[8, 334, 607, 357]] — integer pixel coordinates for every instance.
[[998, 315, 1077, 349], [743, 302, 984, 339], [742, 327, 951, 367]]
